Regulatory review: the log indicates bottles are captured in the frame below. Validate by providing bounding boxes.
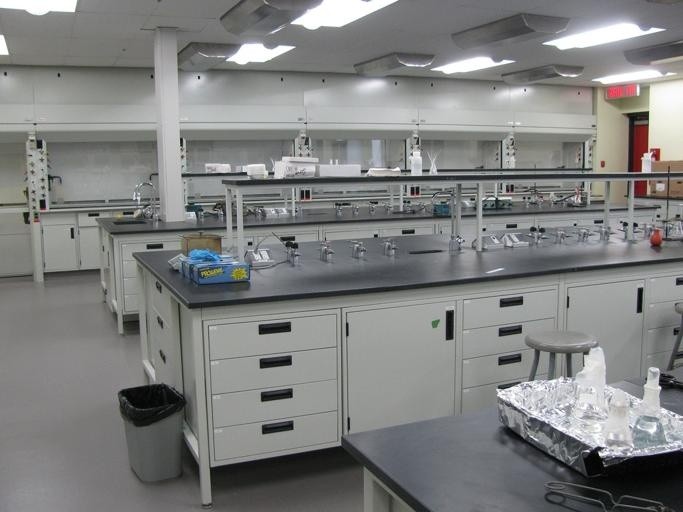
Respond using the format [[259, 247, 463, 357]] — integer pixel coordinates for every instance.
[[505, 184, 509, 192], [300, 186, 303, 200], [640, 152, 653, 175], [596, 392, 631, 451], [510, 183, 514, 192], [415, 185, 418, 195], [629, 365, 668, 447], [410, 184, 414, 195], [305, 187, 309, 200], [649, 227, 662, 247], [410, 150, 422, 175], [572, 346, 608, 414]]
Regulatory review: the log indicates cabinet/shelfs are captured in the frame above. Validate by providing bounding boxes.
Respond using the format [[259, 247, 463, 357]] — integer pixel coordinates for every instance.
[[198, 305, 344, 457], [141, 274, 171, 379], [457, 288, 557, 415], [648, 271, 680, 381], [567, 282, 644, 389], [344, 300, 456, 438], [26, 154, 680, 339]]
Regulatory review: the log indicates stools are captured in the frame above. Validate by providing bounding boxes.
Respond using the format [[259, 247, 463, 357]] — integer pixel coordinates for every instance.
[[669, 301, 682, 373], [523, 330, 599, 381]]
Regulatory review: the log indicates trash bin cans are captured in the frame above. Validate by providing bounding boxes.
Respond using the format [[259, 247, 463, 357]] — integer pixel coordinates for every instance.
[[118, 384, 186, 484]]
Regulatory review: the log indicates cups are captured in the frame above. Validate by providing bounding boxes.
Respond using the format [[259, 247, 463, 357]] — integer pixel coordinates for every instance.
[[235, 165, 240, 172], [264, 170, 268, 178], [242, 165, 247, 172]]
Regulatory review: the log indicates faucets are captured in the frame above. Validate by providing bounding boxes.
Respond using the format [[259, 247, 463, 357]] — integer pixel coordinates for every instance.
[[135, 181, 157, 216], [132, 184, 153, 210]]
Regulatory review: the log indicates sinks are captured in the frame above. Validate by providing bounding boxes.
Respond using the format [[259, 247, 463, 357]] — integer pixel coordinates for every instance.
[[148, 218, 162, 222], [113, 218, 147, 225]]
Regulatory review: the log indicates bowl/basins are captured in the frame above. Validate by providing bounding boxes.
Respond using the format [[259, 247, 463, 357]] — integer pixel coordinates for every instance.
[[244, 164, 266, 180]]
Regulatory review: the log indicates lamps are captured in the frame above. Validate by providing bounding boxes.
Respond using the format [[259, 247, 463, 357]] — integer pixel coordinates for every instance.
[[0, 0, 683, 89]]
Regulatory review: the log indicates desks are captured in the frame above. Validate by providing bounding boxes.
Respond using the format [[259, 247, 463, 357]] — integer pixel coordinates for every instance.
[[337, 388, 683, 511]]
